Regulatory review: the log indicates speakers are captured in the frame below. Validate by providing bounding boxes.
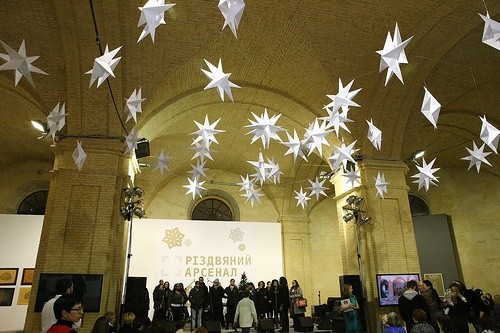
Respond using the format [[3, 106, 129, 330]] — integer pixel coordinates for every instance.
[[339, 275, 362, 303]]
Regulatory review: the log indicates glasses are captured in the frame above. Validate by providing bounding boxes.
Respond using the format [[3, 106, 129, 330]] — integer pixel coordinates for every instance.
[[67, 306, 84, 314]]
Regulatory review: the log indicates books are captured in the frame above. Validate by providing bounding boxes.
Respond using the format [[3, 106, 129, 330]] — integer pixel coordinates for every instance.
[[341, 299, 354, 312]]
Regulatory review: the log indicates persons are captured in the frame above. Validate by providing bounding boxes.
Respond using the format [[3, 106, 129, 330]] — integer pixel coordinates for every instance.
[[41, 277, 85, 333], [275, 276, 290, 333], [90, 275, 308, 333], [233, 289, 259, 333], [340, 284, 360, 333], [382, 279, 500, 333]]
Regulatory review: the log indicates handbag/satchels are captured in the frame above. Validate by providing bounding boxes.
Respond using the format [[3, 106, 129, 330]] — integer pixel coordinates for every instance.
[[166, 311, 173, 322], [295, 294, 308, 308]]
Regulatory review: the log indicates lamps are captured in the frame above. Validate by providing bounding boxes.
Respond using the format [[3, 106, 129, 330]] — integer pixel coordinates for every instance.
[[343, 156, 362, 173], [121, 186, 146, 221], [134, 140, 151, 158], [404, 149, 425, 163], [342, 195, 371, 227], [31, 120, 50, 133], [320, 171, 334, 179]]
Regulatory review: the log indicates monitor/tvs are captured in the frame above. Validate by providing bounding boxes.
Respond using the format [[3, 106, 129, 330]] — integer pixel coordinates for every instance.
[[376, 273, 419, 306], [34, 272, 103, 312]]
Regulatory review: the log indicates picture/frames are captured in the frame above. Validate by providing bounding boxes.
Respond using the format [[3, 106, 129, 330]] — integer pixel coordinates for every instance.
[[0, 288, 15, 306], [21, 268, 35, 285], [424, 273, 446, 297], [0, 268, 19, 285]]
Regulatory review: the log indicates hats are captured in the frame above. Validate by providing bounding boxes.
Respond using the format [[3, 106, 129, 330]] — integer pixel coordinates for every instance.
[[214, 278, 219, 283]]
